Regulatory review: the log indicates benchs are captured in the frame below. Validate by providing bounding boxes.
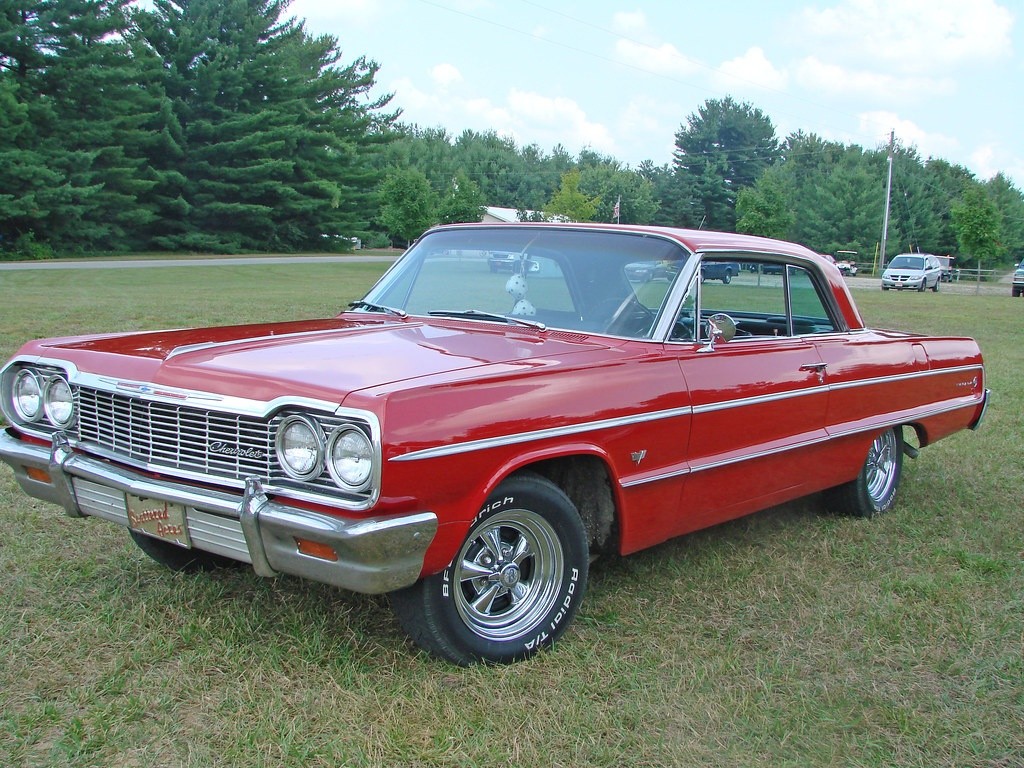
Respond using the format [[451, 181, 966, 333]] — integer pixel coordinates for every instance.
[[678, 317, 817, 337]]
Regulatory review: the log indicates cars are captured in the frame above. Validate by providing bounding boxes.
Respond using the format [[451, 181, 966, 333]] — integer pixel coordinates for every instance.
[[1011, 259, 1024, 297], [487, 250, 514, 273], [881, 253, 942, 293], [665, 258, 742, 286], [624, 261, 668, 282], [761, 262, 796, 275]]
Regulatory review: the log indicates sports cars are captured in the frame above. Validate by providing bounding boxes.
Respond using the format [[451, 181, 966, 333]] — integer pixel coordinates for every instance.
[[0, 222, 991, 668]]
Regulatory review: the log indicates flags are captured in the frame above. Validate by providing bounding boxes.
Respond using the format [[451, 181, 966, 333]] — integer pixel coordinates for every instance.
[[613, 202, 619, 219]]
